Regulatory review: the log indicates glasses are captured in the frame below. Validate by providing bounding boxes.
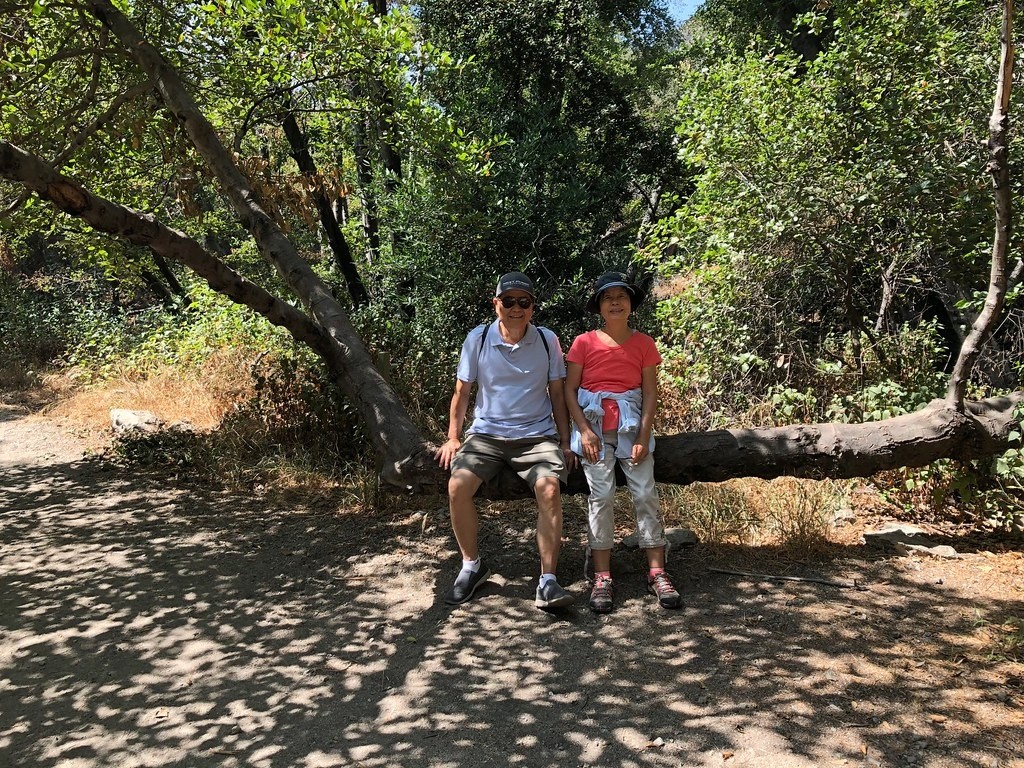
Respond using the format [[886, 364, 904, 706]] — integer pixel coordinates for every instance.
[[497, 296, 532, 309]]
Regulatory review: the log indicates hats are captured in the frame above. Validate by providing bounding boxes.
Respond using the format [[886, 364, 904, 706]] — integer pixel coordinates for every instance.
[[585, 271, 645, 314], [496, 272, 533, 297]]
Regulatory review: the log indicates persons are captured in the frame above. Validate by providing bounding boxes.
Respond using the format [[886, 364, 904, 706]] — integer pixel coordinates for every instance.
[[431, 271, 578, 608], [565, 272, 682, 612]]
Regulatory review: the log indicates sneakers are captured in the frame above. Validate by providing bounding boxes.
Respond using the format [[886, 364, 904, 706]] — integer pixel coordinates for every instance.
[[443, 560, 491, 605], [535, 579, 574, 607], [588, 573, 614, 613], [646, 571, 681, 605]]
